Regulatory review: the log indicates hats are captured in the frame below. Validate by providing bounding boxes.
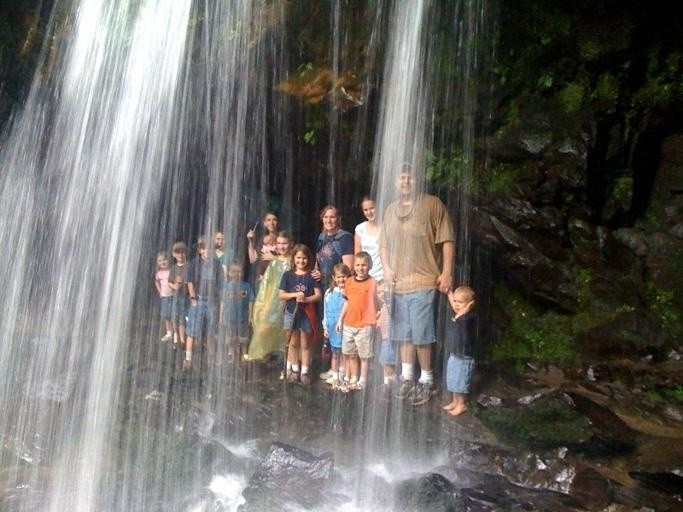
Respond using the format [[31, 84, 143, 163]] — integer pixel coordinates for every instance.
[[172, 241, 188, 251], [189, 235, 216, 247], [394, 162, 414, 169]]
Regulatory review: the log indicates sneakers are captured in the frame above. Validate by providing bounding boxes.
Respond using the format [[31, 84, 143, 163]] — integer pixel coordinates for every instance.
[[158, 332, 441, 406]]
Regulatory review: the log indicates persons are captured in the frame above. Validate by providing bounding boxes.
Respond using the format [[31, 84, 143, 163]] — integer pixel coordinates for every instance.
[[247, 208, 322, 386], [312, 196, 385, 391], [443, 286, 476, 416], [378, 162, 455, 407], [154, 230, 254, 370]]
